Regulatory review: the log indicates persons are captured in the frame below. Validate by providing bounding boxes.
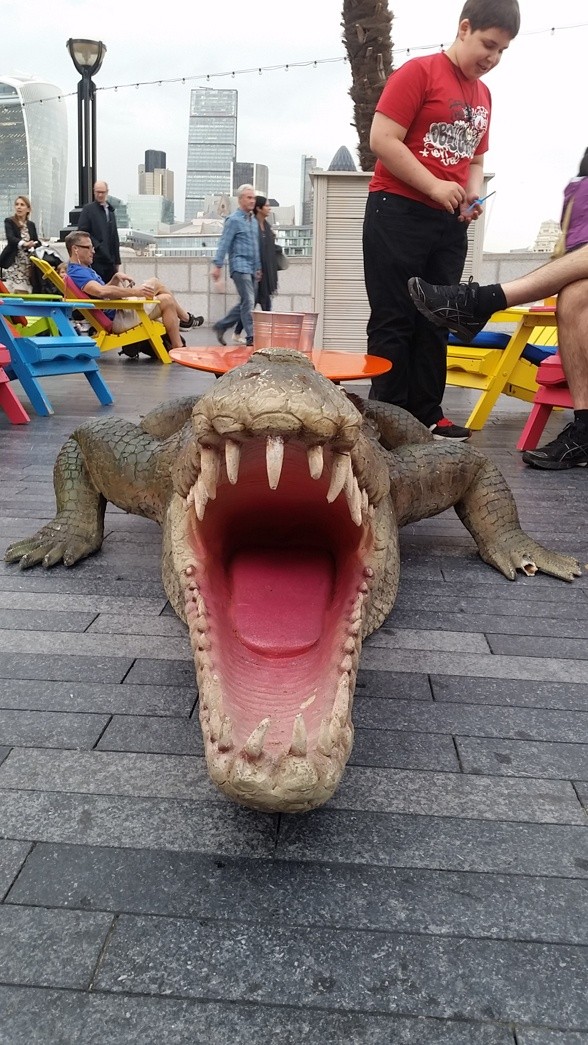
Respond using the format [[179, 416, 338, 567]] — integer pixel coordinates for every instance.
[[0, 195, 42, 285], [362, 0, 521, 442], [57, 262, 69, 278], [78, 182, 121, 284], [212, 184, 277, 346], [65, 231, 204, 349], [408, 147, 588, 470]]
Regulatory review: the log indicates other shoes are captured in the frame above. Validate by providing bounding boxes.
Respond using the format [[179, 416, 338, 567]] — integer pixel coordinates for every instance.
[[212, 322, 227, 345], [232, 332, 246, 345], [246, 335, 254, 347]]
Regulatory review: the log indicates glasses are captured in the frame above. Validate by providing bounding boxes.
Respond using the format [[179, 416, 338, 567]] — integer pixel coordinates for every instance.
[[74, 245, 95, 250]]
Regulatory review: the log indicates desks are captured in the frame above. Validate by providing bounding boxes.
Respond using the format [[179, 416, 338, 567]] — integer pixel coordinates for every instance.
[[165, 346, 392, 385]]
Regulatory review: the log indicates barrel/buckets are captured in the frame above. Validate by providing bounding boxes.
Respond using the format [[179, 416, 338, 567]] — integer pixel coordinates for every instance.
[[250, 311, 305, 353], [284, 311, 320, 352]]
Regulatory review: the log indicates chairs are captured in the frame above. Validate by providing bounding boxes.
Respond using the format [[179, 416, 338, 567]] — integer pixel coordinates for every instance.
[[517, 306, 588, 455], [446, 295, 560, 430], [0, 255, 172, 425]]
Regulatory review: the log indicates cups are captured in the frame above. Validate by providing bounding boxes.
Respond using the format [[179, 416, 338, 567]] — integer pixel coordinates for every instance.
[[144, 280, 154, 300]]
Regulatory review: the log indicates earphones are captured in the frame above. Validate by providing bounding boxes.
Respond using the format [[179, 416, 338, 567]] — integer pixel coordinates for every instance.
[[75, 249, 77, 252]]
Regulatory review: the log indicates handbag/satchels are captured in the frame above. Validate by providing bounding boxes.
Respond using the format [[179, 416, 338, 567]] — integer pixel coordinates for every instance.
[[550, 231, 566, 257]]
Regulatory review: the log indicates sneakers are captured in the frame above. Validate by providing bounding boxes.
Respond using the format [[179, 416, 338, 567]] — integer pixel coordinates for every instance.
[[179, 312, 204, 331], [420, 405, 472, 442], [408, 275, 492, 345], [522, 418, 588, 470]]
